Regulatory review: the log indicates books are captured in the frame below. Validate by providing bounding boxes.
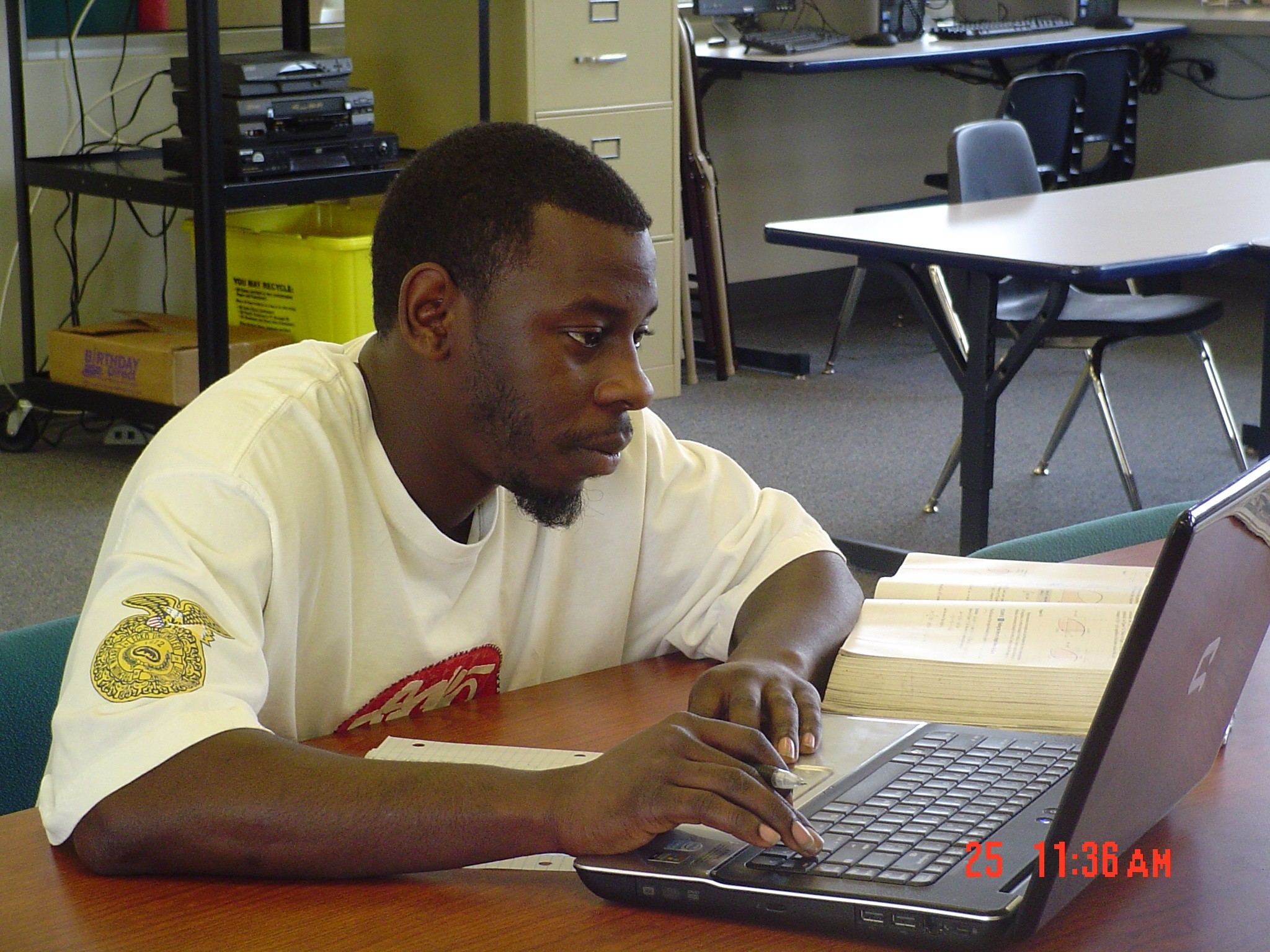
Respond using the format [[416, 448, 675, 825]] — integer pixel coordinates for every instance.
[[820, 549, 1156, 737]]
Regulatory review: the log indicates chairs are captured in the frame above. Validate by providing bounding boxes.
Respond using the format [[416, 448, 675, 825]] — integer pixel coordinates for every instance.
[[825, 40, 1140, 376], [923, 118, 1247, 514]]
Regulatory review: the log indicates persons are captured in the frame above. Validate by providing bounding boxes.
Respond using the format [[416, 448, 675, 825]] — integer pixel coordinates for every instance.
[[38, 122, 864, 888]]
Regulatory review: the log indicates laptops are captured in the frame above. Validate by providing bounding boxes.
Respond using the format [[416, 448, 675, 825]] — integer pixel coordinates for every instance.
[[571, 455, 1269, 951]]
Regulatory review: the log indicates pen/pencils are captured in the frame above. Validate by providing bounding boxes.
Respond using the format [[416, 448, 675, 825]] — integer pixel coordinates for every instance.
[[753, 762, 807, 789]]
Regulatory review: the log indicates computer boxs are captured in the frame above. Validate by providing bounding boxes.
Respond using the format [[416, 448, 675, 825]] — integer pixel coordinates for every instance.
[[758, 0, 926, 43], [953, 0, 1118, 28]]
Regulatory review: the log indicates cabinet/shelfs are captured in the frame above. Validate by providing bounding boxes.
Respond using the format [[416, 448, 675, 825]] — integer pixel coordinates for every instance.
[[342, 0, 686, 401], [6, 0, 496, 438]]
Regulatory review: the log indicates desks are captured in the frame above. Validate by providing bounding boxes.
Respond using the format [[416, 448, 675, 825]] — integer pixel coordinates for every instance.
[[0, 533, 1270, 952], [684, 24, 1194, 374], [763, 159, 1270, 555]]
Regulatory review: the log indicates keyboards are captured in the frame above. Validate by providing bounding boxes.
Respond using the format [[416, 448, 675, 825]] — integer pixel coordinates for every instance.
[[930, 14, 1075, 39], [739, 24, 853, 56]]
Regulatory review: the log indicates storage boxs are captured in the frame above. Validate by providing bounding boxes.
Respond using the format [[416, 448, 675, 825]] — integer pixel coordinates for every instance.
[[170, 191, 389, 348], [47, 310, 290, 407]]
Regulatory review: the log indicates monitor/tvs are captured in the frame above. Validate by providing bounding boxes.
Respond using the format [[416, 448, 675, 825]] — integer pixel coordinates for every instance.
[[692, 0, 796, 47]]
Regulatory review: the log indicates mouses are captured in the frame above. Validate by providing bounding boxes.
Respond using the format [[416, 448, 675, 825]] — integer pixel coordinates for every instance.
[[856, 32, 899, 47], [1105, 16, 1134, 29]]
